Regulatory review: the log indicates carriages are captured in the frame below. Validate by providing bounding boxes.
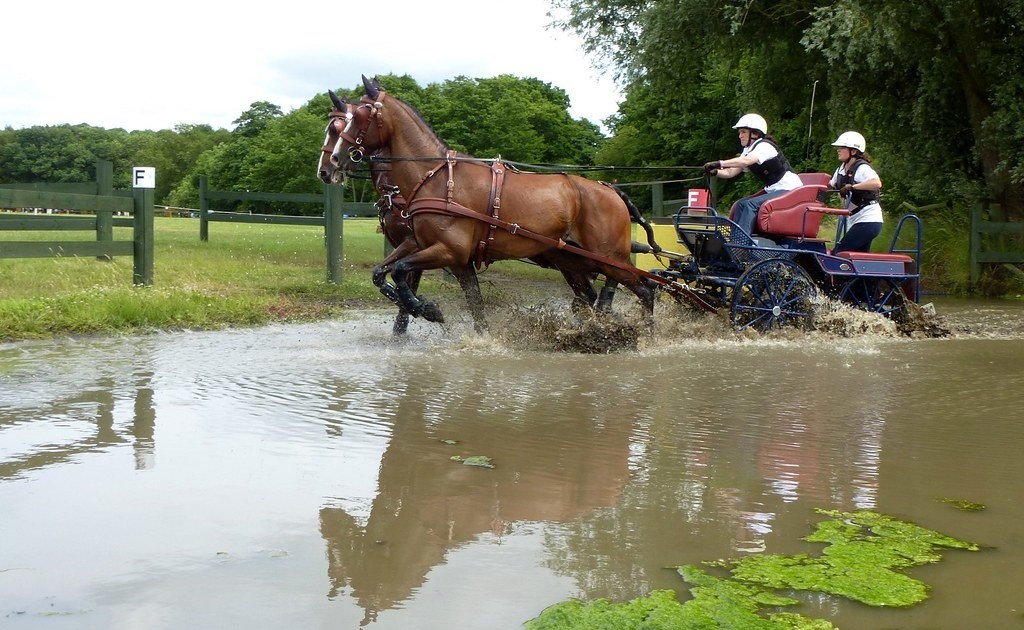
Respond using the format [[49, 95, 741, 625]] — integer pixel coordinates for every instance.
[[317, 75, 923, 338]]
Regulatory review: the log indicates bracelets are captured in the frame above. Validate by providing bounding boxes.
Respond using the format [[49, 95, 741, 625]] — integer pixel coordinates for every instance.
[[719, 159, 725, 170]]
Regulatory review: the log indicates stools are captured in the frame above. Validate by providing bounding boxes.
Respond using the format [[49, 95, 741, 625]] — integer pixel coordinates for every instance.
[[837, 252, 913, 267]]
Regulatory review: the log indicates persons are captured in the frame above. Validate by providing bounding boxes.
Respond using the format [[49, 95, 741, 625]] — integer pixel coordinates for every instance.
[[9, 207, 129, 217], [702, 113, 804, 276], [825, 130, 883, 256]]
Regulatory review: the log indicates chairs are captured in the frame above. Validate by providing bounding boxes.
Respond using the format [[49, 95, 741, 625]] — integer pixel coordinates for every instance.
[[729, 172, 832, 237]]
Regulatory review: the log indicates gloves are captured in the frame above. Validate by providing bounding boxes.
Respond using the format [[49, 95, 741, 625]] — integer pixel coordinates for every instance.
[[702, 162, 720, 171], [704, 169, 717, 177], [839, 184, 853, 199]]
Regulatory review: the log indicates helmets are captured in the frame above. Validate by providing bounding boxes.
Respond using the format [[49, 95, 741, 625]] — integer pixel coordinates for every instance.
[[831, 130, 866, 153], [732, 113, 770, 136]]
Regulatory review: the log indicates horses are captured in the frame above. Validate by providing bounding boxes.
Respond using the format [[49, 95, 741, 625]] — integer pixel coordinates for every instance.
[[315, 74, 656, 344]]
